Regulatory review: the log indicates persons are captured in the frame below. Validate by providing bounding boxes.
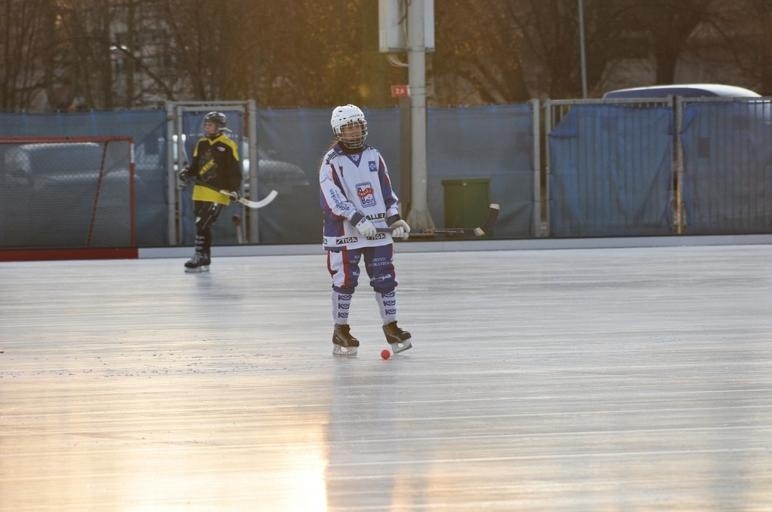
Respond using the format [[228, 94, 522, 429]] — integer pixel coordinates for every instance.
[[177, 111, 244, 271], [315, 103, 413, 354]]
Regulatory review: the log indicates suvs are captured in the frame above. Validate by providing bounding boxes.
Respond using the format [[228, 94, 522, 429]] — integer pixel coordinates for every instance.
[[131, 129, 308, 192]]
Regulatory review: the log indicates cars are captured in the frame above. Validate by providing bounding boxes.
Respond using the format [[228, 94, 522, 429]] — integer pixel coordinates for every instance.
[[2, 142, 146, 247]]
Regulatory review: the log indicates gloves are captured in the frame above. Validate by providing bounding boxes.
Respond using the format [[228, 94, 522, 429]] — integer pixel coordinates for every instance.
[[386, 213, 411, 241], [178, 163, 194, 182], [349, 211, 376, 238], [229, 185, 242, 201]]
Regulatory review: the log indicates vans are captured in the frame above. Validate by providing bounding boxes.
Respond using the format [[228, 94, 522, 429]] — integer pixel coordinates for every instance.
[[603, 82, 772, 125]]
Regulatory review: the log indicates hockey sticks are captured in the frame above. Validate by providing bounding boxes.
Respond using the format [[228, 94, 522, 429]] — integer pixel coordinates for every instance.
[[184, 176, 277, 210], [375, 204, 500, 234]]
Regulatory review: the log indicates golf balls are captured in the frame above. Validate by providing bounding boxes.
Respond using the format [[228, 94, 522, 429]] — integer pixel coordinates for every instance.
[[381, 349, 389, 359]]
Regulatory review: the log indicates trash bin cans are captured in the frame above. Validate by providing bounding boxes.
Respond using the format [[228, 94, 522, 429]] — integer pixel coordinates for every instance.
[[440, 178, 490, 240]]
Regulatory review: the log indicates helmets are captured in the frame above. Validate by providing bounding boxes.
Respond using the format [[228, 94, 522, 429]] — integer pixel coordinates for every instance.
[[201, 111, 228, 139], [331, 104, 369, 150]]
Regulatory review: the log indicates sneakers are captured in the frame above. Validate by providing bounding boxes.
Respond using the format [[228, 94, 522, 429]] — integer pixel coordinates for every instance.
[[332, 323, 359, 347], [184, 249, 210, 267], [382, 321, 411, 343]]
